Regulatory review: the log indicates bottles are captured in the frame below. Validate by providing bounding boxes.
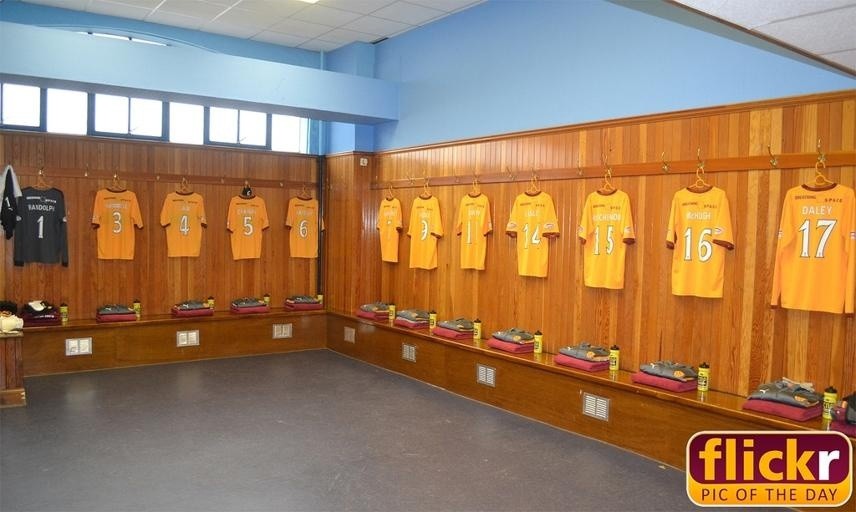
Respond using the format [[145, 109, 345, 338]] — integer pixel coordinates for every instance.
[[428, 311, 437, 331], [473, 319, 482, 338], [534, 331, 543, 354], [317, 291, 324, 304], [697, 363, 710, 393], [208, 296, 214, 308], [60, 302, 69, 323], [825, 386, 838, 419], [133, 299, 141, 317], [388, 302, 397, 323], [264, 294, 270, 305], [609, 346, 619, 370]]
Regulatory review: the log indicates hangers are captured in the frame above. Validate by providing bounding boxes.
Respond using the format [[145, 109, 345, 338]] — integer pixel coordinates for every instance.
[[384, 158, 837, 192], [28, 168, 314, 200]]
